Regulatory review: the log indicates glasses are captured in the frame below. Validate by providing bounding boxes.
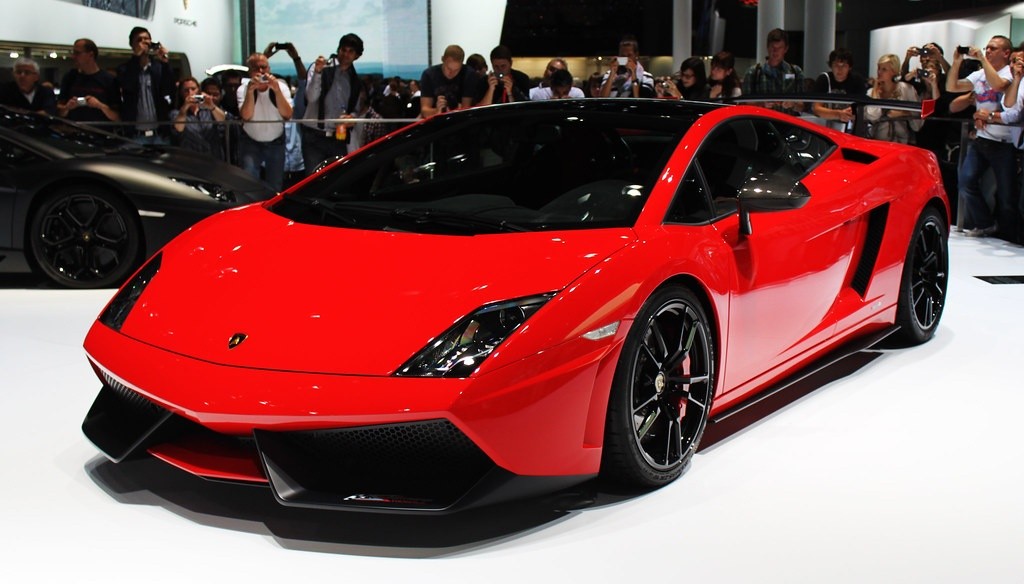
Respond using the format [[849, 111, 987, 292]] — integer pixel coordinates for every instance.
[[680, 72, 695, 79]]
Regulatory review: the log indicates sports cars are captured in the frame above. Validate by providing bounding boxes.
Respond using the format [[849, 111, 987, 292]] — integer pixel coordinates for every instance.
[[0, 104, 287, 290], [81, 99, 951, 520]]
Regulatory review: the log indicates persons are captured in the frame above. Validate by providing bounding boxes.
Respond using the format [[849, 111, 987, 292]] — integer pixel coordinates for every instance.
[[0, 27, 1024, 248]]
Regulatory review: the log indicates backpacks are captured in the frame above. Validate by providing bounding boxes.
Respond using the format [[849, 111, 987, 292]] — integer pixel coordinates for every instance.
[[293, 59, 336, 120]]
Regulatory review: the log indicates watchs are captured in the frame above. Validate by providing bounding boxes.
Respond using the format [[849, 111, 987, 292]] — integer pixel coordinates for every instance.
[[988, 112, 994, 119]]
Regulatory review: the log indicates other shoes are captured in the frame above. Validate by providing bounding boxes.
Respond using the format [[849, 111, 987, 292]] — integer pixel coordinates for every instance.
[[965, 225, 997, 236]]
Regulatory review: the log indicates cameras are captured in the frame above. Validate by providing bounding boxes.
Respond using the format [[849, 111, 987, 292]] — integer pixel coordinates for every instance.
[[323, 59, 332, 65], [258, 75, 270, 83], [76, 97, 86, 104], [660, 81, 669, 87], [193, 95, 204, 103]]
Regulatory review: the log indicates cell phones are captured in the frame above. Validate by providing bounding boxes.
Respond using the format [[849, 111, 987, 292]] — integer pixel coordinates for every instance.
[[958, 47, 969, 54], [492, 73, 504, 78], [614, 57, 628, 65], [916, 69, 929, 77], [146, 42, 159, 49], [916, 48, 928, 55]]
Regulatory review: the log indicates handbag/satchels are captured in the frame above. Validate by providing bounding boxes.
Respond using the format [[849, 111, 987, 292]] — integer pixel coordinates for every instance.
[[801, 72, 846, 132]]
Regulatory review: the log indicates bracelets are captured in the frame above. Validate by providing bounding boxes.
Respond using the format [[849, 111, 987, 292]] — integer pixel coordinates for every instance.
[[211, 107, 215, 111], [507, 93, 512, 96], [163, 54, 168, 58], [632, 79, 639, 84]]
